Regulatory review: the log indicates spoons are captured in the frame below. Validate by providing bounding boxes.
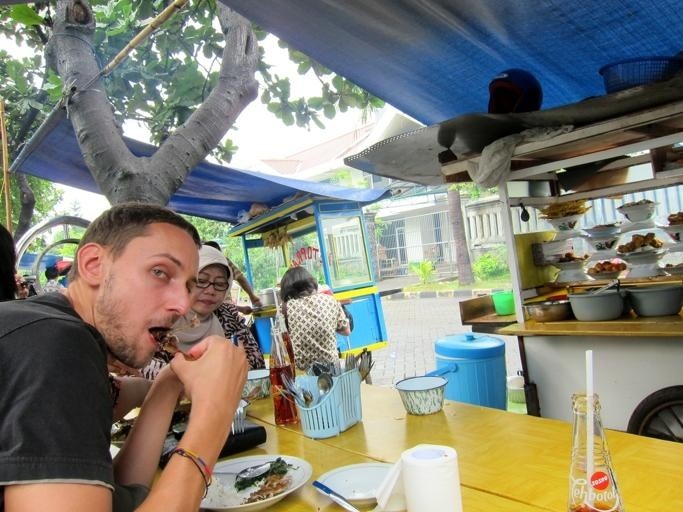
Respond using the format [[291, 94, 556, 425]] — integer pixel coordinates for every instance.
[[316, 372, 334, 403]]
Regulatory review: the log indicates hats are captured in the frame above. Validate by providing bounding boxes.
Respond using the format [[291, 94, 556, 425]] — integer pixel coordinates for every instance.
[[197, 244, 233, 295]]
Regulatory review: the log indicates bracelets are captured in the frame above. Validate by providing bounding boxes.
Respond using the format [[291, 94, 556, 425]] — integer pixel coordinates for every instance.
[[160, 448, 213, 500]]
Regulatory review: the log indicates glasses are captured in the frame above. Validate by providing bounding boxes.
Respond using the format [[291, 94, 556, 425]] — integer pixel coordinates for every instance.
[[194, 277, 229, 293]]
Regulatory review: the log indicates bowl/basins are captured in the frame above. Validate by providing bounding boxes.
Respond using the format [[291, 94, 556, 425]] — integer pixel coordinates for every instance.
[[395, 376, 449, 416], [546, 201, 683, 283], [524, 284, 683, 322], [241, 369, 270, 401]]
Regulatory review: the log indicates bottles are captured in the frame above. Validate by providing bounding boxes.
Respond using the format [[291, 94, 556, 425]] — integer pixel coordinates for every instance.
[[568, 393, 625, 512], [270, 316, 298, 425]]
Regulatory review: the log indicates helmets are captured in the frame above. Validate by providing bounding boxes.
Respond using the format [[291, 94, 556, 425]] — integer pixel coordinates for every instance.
[[488, 68, 542, 114]]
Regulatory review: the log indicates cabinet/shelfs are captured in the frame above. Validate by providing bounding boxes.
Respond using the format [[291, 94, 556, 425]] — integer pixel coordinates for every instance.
[[441, 102, 683, 324]]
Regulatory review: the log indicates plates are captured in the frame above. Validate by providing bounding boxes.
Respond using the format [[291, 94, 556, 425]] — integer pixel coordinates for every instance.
[[200, 453, 396, 511]]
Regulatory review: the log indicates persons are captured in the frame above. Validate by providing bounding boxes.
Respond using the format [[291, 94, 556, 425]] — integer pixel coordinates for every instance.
[[0, 224, 152, 425], [15, 258, 78, 300], [140, 244, 265, 379], [0, 203, 250, 512], [202, 241, 262, 315], [274, 265, 350, 375]]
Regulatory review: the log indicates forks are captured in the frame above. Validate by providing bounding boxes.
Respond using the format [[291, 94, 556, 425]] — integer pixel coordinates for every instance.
[[345, 350, 374, 380], [231, 406, 246, 436]]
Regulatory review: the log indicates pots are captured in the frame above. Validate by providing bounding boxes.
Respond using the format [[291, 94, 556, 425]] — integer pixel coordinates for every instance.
[[259, 288, 283, 308]]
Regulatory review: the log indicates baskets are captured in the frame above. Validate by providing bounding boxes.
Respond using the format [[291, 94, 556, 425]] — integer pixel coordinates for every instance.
[[294, 368, 363, 440], [599, 56, 673, 94]]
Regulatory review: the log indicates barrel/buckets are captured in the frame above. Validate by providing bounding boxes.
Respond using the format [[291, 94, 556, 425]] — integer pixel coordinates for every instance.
[[371, 443, 460, 511], [427, 330, 509, 414]]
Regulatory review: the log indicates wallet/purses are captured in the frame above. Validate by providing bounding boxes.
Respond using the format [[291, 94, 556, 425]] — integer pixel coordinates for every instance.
[[159, 419, 266, 468]]
